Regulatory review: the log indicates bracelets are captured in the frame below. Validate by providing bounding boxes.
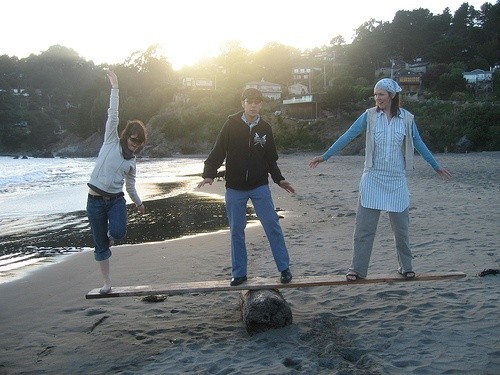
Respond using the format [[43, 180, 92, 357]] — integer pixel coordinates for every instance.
[[137, 204, 142, 207]]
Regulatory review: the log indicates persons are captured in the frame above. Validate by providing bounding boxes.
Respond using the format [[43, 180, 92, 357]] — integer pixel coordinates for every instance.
[[198, 88, 295, 286], [309, 79, 452, 281], [86, 71, 147, 293]]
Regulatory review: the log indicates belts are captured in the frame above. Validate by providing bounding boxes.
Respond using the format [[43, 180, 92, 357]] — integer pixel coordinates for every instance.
[[88, 192, 124, 200]]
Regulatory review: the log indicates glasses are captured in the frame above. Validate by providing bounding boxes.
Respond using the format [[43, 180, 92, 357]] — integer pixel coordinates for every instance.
[[130, 136, 142, 145], [245, 98, 260, 104]]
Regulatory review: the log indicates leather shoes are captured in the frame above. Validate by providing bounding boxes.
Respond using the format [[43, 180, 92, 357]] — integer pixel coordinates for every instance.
[[230, 276, 247, 286], [280, 270, 292, 283]]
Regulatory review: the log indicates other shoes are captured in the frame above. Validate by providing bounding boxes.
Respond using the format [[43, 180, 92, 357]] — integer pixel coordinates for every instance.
[[99, 283, 112, 294]]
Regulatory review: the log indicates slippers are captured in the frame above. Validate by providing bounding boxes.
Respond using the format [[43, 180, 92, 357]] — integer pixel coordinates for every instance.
[[398, 268, 415, 278], [346, 271, 359, 281]]
